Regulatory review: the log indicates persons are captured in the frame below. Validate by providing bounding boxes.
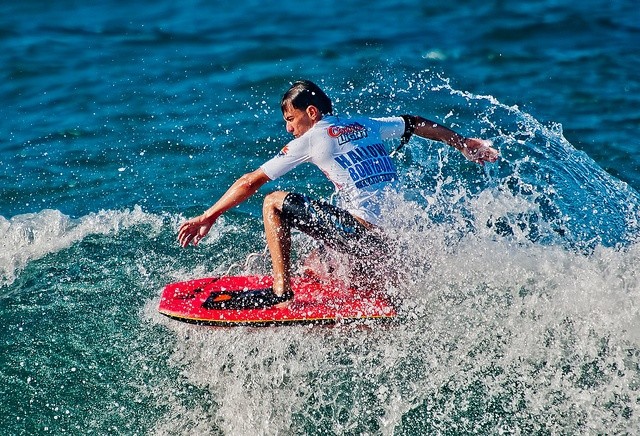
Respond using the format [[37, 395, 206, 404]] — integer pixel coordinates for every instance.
[[178, 81, 500, 309]]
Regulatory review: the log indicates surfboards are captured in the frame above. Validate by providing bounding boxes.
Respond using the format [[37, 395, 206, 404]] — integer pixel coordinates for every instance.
[[158, 274, 398, 324]]
